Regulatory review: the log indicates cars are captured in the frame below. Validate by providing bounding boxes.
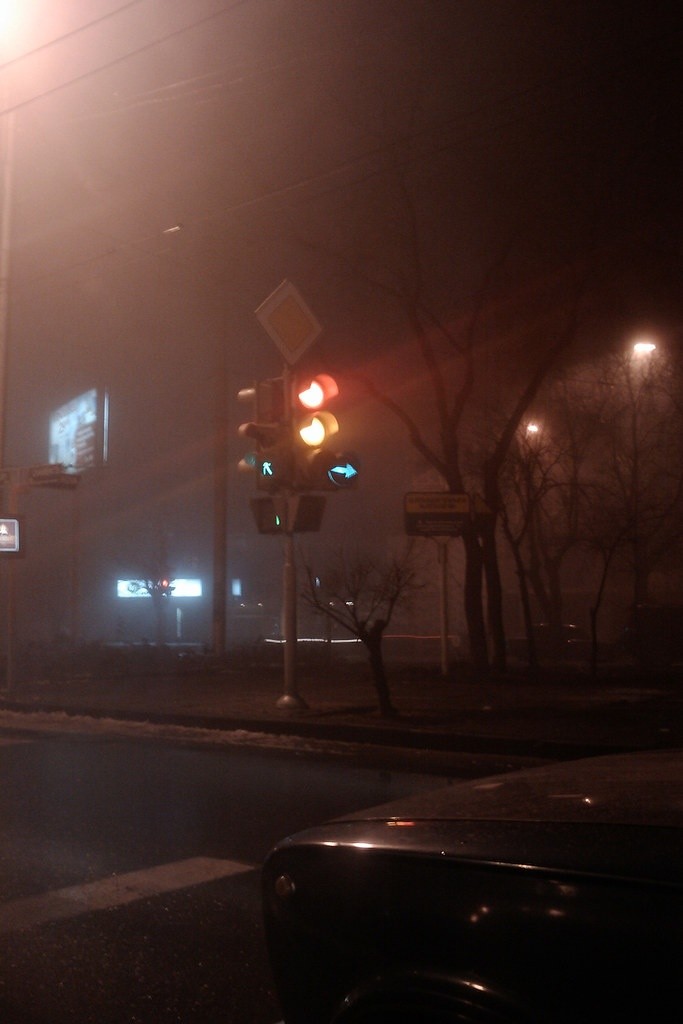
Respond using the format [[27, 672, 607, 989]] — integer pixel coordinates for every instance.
[[255, 745, 683, 1024]]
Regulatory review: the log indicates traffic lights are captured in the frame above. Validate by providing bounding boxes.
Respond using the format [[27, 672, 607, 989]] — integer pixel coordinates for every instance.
[[286, 363, 360, 487], [245, 376, 283, 491], [287, 495, 328, 534], [249, 496, 287, 534]]
